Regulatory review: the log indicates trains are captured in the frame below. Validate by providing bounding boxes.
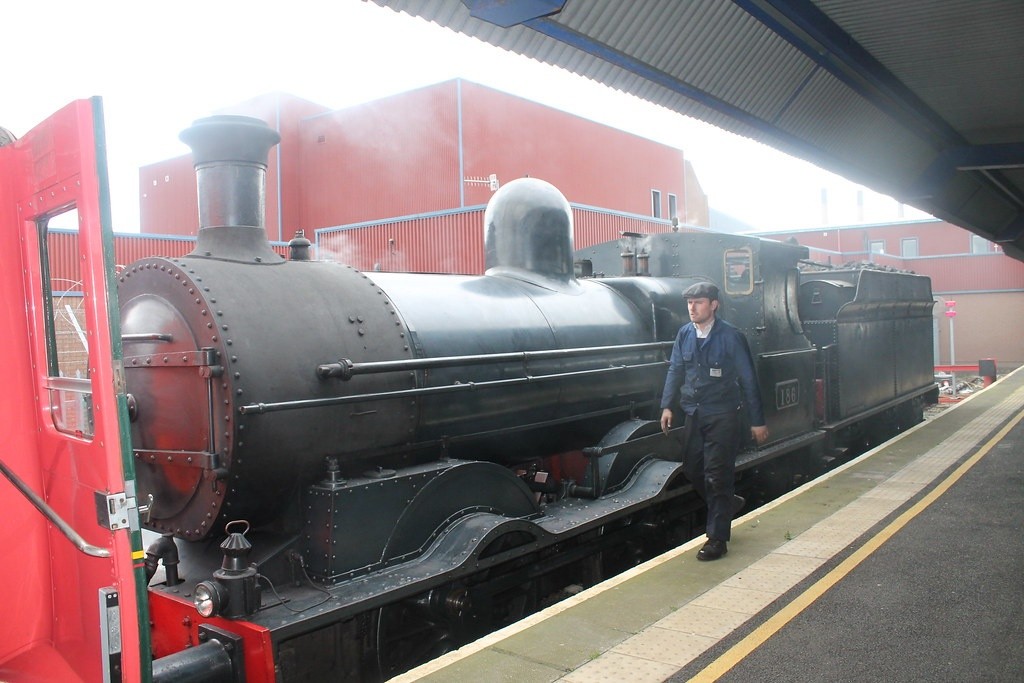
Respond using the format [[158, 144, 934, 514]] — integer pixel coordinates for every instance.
[[0, 72, 940, 683]]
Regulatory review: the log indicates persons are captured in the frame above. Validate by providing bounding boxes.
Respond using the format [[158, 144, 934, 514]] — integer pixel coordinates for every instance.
[[661, 282, 769, 560]]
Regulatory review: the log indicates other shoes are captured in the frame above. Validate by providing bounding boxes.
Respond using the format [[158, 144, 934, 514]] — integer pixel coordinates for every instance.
[[733, 495, 745, 514], [695, 539, 728, 559]]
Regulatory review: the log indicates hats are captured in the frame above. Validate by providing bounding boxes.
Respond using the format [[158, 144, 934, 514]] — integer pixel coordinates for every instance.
[[682, 282, 720, 298]]
